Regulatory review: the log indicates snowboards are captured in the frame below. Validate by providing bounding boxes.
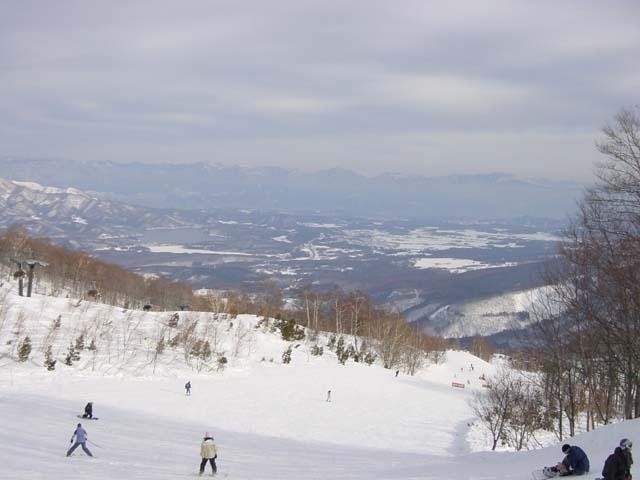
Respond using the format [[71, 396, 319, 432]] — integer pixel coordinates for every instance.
[[76, 414, 99, 419], [531, 465, 559, 479]]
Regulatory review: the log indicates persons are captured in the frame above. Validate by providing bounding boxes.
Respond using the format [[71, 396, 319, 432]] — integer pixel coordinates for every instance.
[[199, 430, 219, 476], [82, 402, 93, 419], [543, 442, 590, 475], [395, 370, 400, 377], [600, 437, 634, 480], [325, 389, 332, 403], [185, 381, 192, 395], [66, 423, 95, 458]]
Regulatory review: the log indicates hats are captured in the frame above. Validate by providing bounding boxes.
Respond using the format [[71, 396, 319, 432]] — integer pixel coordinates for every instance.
[[620, 439, 632, 450]]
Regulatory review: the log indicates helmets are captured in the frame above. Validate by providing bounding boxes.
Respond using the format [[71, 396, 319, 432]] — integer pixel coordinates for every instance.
[[543, 466, 558, 477]]
